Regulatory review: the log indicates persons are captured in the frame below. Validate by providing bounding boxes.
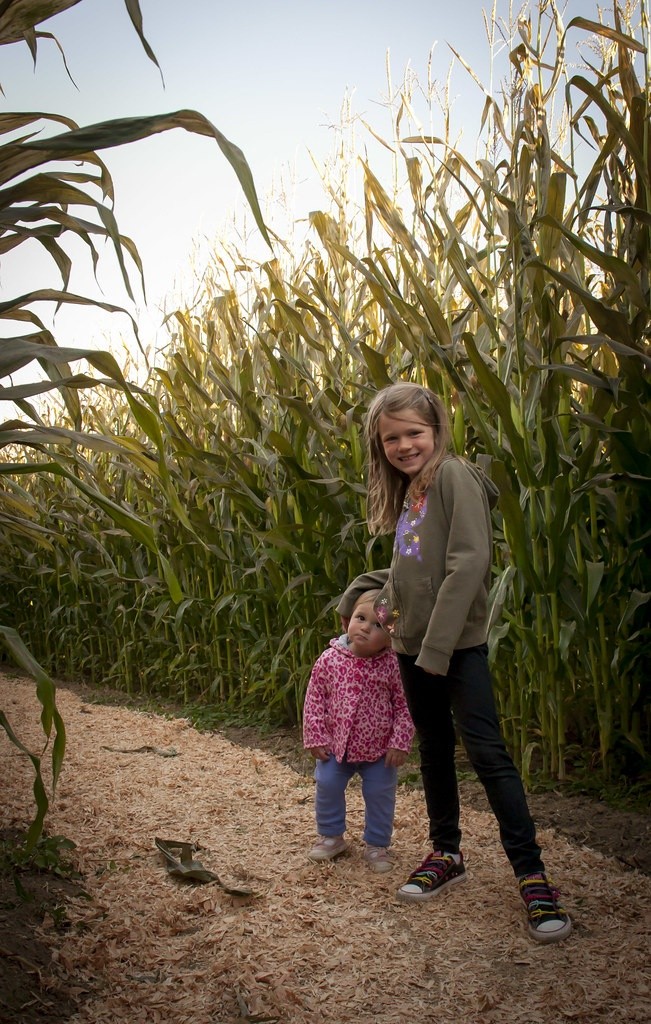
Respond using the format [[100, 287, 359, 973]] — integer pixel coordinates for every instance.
[[301, 589, 416, 873], [334, 381, 572, 944]]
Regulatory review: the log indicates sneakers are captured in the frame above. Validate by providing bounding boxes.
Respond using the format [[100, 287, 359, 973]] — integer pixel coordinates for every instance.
[[397, 849, 467, 899], [519, 872, 574, 942]]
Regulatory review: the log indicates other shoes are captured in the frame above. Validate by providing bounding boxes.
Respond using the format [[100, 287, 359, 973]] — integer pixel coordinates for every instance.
[[309, 836, 350, 860], [361, 844, 396, 874]]
